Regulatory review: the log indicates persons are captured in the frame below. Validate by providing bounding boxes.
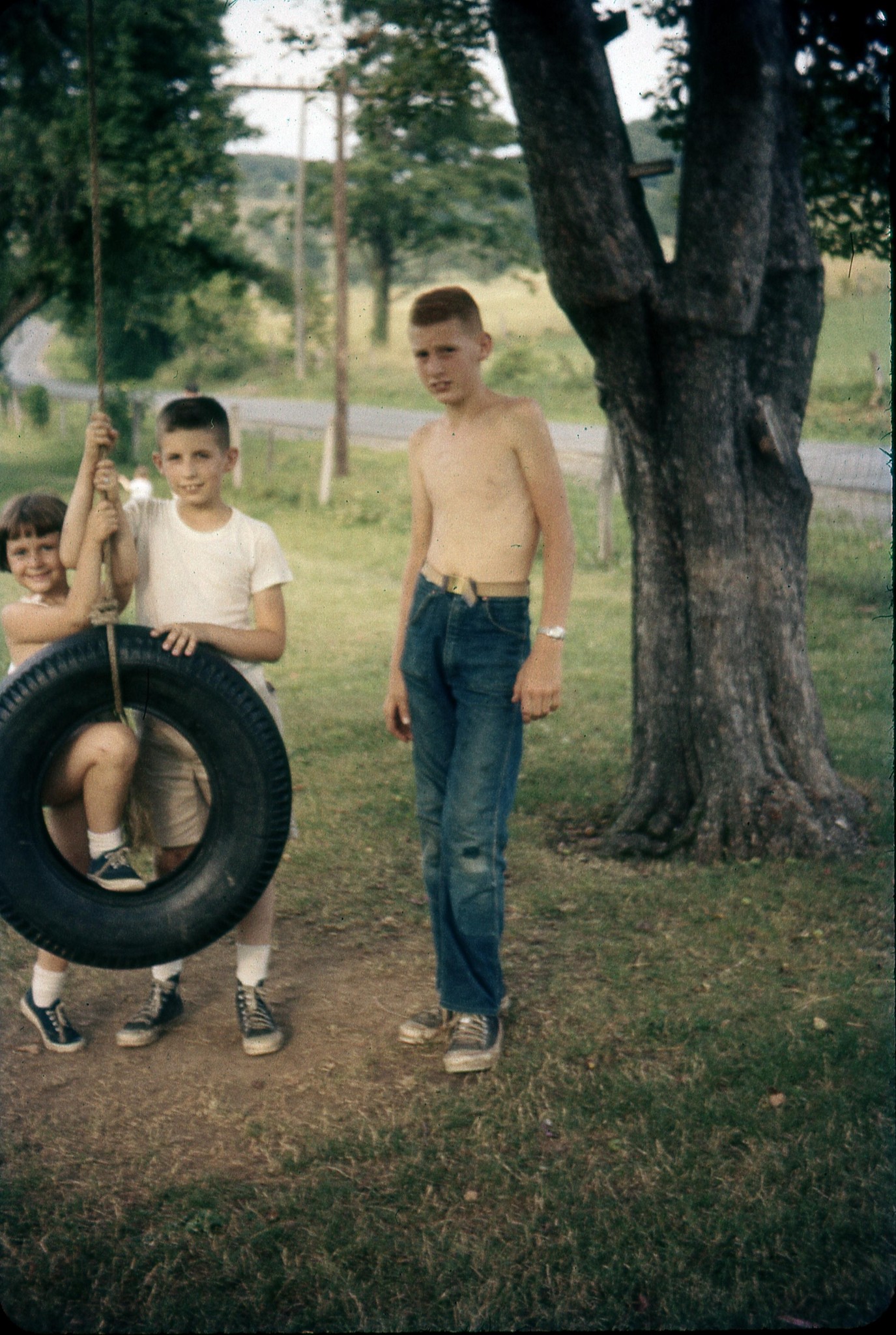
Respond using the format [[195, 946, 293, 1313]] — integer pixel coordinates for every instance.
[[0, 459, 146, 1055], [118, 462, 152, 518], [363, 278, 575, 1082], [57, 388, 289, 1057]]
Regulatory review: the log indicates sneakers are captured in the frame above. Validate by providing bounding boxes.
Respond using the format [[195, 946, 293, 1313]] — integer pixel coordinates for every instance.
[[236, 977, 284, 1057], [113, 971, 186, 1047], [20, 987, 86, 1053], [442, 1012, 503, 1073], [395, 1004, 457, 1045], [84, 845, 146, 892]]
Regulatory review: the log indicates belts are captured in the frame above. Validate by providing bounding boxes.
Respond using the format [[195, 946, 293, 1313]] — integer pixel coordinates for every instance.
[[419, 560, 530, 608]]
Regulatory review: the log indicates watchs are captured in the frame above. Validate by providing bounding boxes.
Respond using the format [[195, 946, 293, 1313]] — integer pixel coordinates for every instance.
[[533, 623, 569, 644]]
